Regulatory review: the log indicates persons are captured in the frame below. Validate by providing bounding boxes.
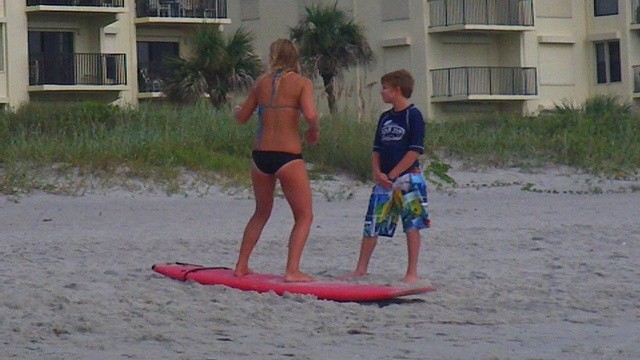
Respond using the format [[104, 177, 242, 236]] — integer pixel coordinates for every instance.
[[338, 68, 431, 283], [232, 38, 322, 282]]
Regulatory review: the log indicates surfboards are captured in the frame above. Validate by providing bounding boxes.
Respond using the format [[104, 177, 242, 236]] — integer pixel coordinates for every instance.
[[152, 261, 437, 301]]
